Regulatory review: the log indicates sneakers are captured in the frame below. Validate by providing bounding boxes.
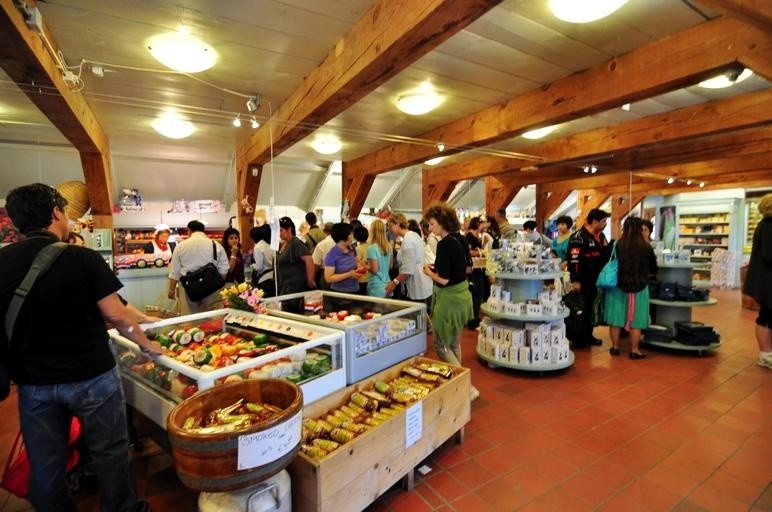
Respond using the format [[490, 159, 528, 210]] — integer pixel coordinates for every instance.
[[756, 353, 772, 370], [470, 386, 479, 404], [130, 500, 150, 512]]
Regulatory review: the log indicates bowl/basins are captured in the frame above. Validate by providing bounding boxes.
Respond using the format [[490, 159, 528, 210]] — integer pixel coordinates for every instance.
[[166, 377, 303, 492]]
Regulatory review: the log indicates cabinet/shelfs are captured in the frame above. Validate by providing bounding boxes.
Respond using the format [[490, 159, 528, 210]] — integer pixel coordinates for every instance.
[[114, 224, 228, 255], [640, 262, 722, 355], [475, 271, 576, 372], [676, 197, 742, 286]]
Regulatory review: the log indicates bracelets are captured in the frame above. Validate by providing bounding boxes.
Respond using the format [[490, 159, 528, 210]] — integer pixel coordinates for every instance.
[[140, 339, 151, 353], [169, 288, 175, 293], [137, 313, 146, 323]]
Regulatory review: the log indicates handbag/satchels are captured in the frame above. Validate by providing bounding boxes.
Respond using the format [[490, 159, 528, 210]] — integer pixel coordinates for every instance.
[[468, 280, 481, 296], [562, 290, 586, 339], [180, 263, 225, 301], [596, 239, 618, 288]]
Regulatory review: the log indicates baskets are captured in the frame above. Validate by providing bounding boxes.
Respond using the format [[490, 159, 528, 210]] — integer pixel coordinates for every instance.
[[199, 287, 232, 313], [146, 295, 180, 319]]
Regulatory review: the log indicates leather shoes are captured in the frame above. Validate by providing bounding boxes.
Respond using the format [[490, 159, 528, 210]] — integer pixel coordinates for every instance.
[[629, 352, 648, 358], [610, 347, 620, 355]]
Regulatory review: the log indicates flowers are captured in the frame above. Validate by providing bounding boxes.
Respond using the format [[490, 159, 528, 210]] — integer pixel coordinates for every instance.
[[221, 282, 269, 313]]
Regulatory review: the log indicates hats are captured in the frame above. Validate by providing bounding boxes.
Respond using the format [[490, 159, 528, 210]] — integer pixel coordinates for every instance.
[[154, 224, 170, 235], [759, 194, 772, 214], [587, 208, 611, 222]]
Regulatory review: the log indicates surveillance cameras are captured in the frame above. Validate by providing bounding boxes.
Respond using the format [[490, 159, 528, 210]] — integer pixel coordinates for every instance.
[[245, 204, 255, 214]]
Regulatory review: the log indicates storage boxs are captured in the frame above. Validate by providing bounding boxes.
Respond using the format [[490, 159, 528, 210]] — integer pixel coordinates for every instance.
[[291, 355, 472, 511]]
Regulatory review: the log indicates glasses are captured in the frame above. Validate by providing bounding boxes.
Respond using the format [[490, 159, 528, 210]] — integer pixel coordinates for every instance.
[[279, 218, 287, 222], [36, 182, 55, 205]]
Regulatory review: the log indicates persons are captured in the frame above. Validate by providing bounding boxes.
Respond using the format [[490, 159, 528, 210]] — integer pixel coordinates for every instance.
[[120, 188, 132, 205], [218, 207, 658, 360], [0, 183, 164, 511], [131, 189, 142, 206], [741, 193, 772, 369], [63, 231, 165, 324], [142, 224, 177, 263], [167, 220, 230, 316], [421, 201, 480, 403]]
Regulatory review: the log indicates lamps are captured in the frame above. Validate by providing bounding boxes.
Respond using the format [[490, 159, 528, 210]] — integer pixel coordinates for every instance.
[[582, 165, 599, 174], [229, 112, 260, 130], [667, 176, 706, 189], [395, 92, 445, 116], [433, 142, 446, 152], [144, 32, 221, 75]]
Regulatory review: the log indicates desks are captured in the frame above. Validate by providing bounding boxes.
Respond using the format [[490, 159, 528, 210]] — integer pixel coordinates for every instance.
[[105, 305, 349, 461], [259, 285, 430, 387]]
